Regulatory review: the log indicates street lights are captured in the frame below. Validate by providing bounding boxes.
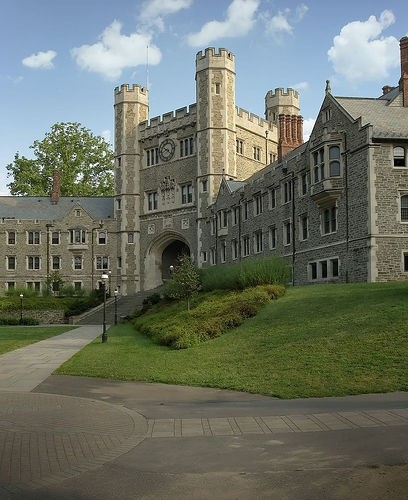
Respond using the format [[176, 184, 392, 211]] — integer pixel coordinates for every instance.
[[114, 289, 118, 326], [101, 271, 108, 344], [19, 294, 24, 324]]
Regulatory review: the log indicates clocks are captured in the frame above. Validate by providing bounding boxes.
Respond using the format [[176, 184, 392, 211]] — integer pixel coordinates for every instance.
[[158, 138, 176, 162]]
